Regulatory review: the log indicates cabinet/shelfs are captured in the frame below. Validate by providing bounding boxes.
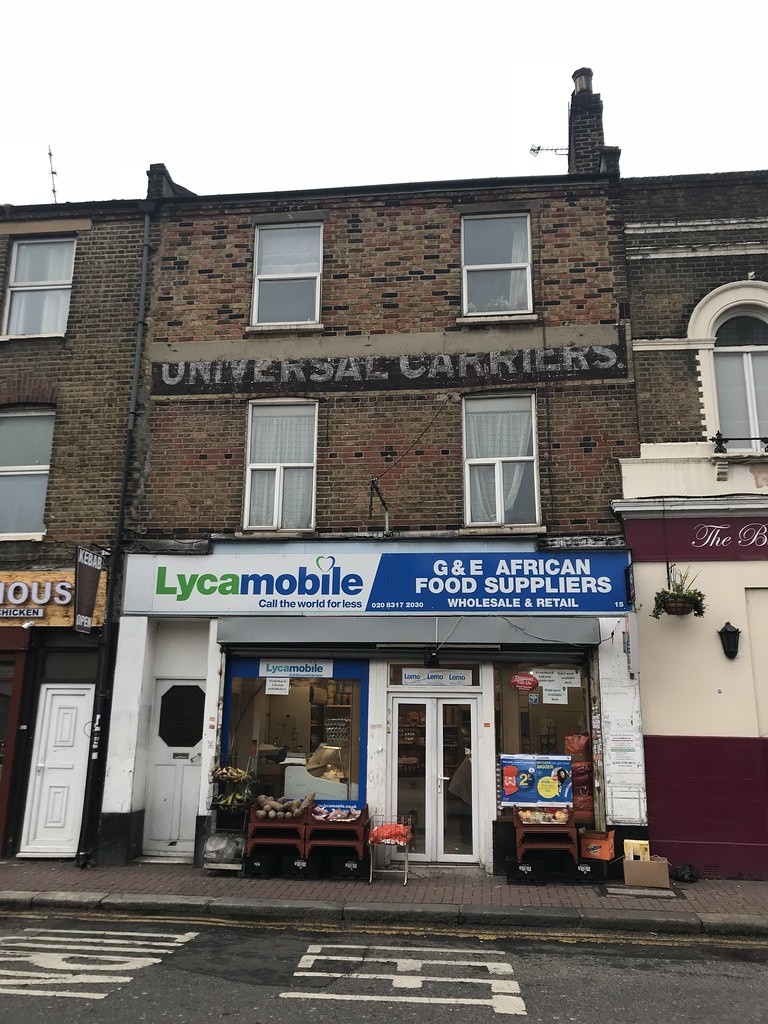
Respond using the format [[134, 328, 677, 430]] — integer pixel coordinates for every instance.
[[245, 800, 371, 859], [513, 806, 579, 864], [322, 703, 461, 768], [202, 756, 254, 878]]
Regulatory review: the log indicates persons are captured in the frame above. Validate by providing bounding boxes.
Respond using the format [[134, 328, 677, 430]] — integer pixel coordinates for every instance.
[[448, 754, 472, 847]]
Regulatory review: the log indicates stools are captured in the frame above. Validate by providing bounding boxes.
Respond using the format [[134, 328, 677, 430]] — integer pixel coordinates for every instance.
[[369, 841, 408, 887]]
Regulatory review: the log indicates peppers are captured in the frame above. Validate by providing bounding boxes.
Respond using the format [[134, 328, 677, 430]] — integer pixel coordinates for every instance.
[[216, 792, 246, 813]]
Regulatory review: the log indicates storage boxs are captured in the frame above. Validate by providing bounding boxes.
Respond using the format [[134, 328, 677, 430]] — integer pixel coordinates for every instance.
[[624, 855, 671, 889], [623, 838, 652, 860], [554, 857, 609, 884], [505, 854, 546, 886], [578, 826, 615, 860]]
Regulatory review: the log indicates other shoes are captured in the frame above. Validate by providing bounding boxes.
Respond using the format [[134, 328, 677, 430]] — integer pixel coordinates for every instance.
[[461, 833, 472, 845]]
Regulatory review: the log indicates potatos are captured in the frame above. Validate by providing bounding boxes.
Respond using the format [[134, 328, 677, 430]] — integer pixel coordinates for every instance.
[[254, 792, 314, 820]]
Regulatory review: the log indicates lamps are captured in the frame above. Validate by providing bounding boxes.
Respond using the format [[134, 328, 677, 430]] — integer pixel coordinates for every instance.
[[716, 621, 742, 659], [21, 621, 35, 629]]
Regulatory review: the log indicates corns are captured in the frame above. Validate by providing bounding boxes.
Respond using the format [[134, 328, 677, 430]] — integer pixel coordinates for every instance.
[[212, 765, 251, 784]]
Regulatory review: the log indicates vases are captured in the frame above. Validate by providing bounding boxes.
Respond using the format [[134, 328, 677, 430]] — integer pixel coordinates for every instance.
[[663, 598, 695, 616]]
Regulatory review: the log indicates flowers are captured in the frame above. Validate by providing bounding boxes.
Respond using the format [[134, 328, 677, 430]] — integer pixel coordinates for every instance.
[[649, 562, 709, 620]]
